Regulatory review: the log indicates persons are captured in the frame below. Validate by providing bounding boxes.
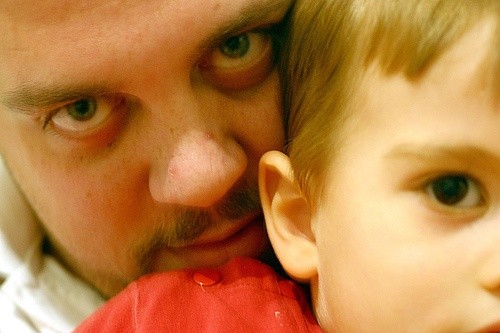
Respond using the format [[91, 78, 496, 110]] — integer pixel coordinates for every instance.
[[1, 1, 289, 331], [69, 0, 500, 332]]
